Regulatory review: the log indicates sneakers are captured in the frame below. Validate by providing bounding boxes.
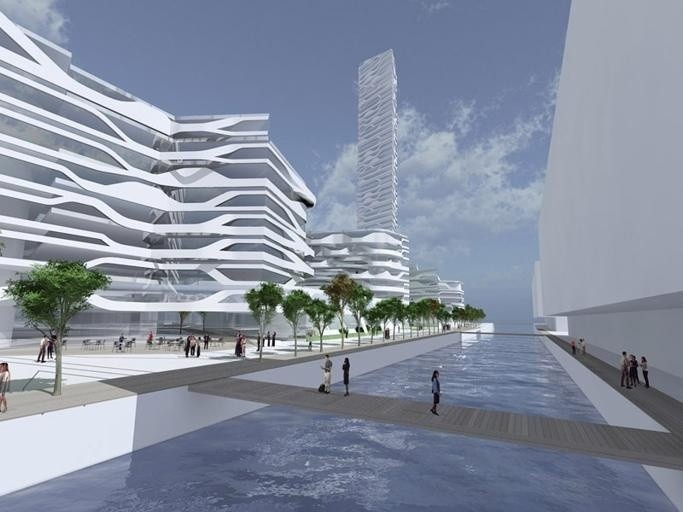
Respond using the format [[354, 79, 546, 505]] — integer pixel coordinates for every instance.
[[630, 383, 637, 387], [41, 361, 46, 363], [626, 386, 632, 389]]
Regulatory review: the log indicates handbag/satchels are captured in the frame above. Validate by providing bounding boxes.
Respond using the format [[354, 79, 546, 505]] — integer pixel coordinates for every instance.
[[318, 383, 325, 392]]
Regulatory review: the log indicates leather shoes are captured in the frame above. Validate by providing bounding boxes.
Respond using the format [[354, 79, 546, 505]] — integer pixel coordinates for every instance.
[[620, 384, 625, 387], [324, 390, 330, 394], [344, 392, 349, 397], [430, 408, 439, 416]]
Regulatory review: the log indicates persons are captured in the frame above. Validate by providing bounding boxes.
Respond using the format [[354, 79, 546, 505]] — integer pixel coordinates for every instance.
[[1, 363, 10, 414], [257, 330, 276, 351], [118, 332, 135, 350], [620, 351, 650, 389], [145, 331, 210, 357], [37, 329, 57, 362], [430, 370, 442, 416], [342, 358, 350, 397], [234, 332, 247, 358], [321, 354, 332, 393], [570, 338, 586, 355]]
